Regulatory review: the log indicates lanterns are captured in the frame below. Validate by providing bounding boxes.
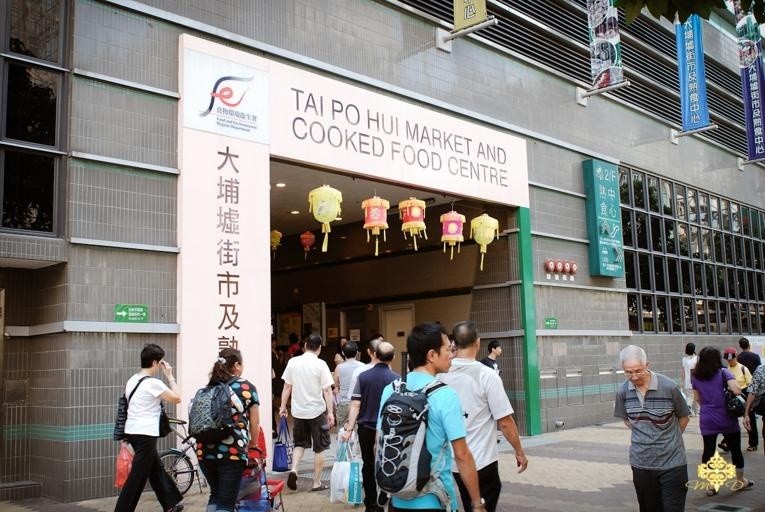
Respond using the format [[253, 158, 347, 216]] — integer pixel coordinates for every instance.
[[469, 213, 499, 270], [398, 196, 428, 249], [308, 185, 343, 252], [270, 229, 282, 258], [441, 211, 466, 260], [300, 230, 314, 258], [361, 195, 388, 252]]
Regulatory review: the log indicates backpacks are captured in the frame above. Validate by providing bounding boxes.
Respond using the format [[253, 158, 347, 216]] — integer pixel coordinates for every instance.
[[372, 373, 451, 512], [190, 375, 235, 443]]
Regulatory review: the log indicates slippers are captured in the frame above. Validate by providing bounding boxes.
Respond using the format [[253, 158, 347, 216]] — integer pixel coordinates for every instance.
[[285, 468, 298, 491], [735, 478, 755, 491], [703, 488, 718, 497], [309, 482, 330, 493]]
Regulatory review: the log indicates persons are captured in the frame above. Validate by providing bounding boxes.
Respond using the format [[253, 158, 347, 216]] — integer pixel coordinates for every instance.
[[115, 344, 184, 512], [331, 342, 370, 461], [680, 343, 700, 415], [691, 346, 754, 497], [189, 347, 261, 512], [613, 345, 691, 512], [287, 333, 301, 361], [742, 364, 765, 452], [738, 337, 761, 376], [293, 338, 305, 357], [434, 321, 528, 512], [719, 347, 758, 451], [481, 341, 503, 375], [374, 322, 487, 512], [340, 342, 403, 512], [271, 338, 285, 413], [333, 337, 346, 367], [278, 332, 334, 491], [347, 339, 392, 426]]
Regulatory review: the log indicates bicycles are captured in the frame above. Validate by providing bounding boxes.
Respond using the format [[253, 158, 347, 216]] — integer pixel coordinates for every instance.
[[157, 417, 207, 492]]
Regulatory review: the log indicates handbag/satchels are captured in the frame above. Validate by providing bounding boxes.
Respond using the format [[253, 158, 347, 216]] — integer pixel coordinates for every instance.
[[269, 416, 292, 472], [329, 439, 364, 509]]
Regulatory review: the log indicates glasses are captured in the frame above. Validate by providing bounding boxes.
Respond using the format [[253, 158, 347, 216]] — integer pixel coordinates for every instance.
[[624, 369, 646, 377]]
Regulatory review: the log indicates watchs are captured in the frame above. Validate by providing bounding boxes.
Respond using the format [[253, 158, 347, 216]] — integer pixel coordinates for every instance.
[[469, 498, 484, 508]]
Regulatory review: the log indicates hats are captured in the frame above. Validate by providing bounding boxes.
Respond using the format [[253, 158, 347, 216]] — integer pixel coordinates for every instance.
[[723, 347, 737, 361]]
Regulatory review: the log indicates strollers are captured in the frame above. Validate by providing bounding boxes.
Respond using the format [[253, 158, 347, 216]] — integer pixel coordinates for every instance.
[[211, 427, 284, 511]]
